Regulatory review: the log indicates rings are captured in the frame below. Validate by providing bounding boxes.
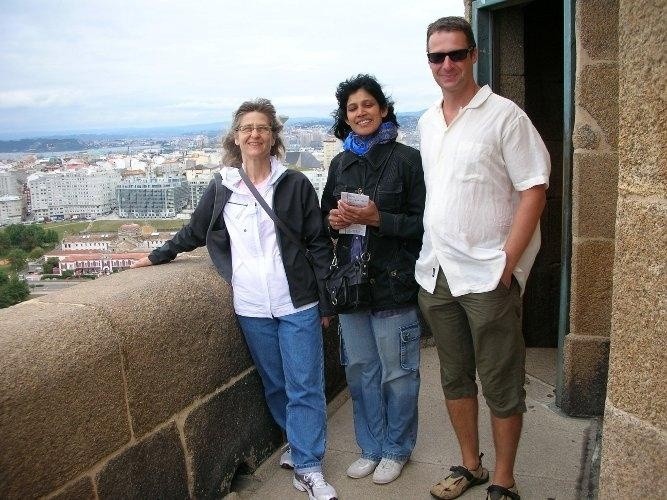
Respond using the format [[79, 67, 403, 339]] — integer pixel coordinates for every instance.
[[335, 222, 337, 225]]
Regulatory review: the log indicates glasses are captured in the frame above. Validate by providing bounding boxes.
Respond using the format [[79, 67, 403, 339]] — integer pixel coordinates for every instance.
[[426, 43, 479, 64], [241, 125, 275, 135]]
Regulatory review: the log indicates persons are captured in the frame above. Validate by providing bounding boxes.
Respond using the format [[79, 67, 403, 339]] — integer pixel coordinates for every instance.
[[130, 95, 342, 500], [403, 14, 556, 500], [320, 76, 428, 486]]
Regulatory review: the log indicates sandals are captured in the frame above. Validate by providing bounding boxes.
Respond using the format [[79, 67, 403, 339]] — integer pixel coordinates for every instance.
[[429, 452, 490, 500], [486, 481, 521, 500]]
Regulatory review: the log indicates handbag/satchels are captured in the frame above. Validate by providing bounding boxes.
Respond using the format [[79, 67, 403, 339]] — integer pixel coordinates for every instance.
[[325, 254, 376, 314]]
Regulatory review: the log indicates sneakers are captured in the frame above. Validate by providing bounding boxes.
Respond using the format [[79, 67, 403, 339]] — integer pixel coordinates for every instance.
[[372, 457, 409, 484], [279, 445, 295, 470], [291, 472, 338, 500], [345, 456, 378, 479]]
